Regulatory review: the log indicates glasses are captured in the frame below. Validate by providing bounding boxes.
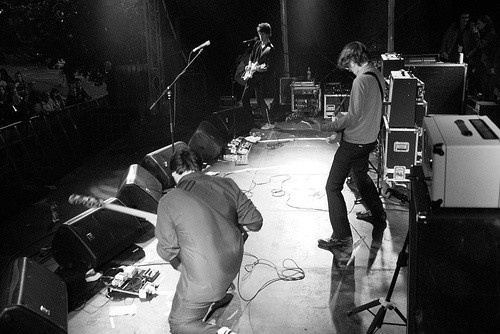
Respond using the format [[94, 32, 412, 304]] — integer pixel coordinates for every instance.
[[344, 59, 354, 70]]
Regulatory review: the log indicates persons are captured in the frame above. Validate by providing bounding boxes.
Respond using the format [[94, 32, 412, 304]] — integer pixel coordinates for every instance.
[[440, 10, 500, 104], [318, 41, 387, 248], [242, 23, 275, 125], [155, 150, 263, 334], [0, 60, 118, 113]]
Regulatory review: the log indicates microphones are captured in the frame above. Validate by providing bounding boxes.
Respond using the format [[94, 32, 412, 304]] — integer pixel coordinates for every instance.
[[243, 37, 258, 43], [192, 40, 211, 52]]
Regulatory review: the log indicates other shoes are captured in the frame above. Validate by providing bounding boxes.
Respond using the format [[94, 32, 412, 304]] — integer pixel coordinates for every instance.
[[318, 237, 352, 247], [261, 123, 275, 129], [356, 211, 386, 221], [216, 326, 237, 334]]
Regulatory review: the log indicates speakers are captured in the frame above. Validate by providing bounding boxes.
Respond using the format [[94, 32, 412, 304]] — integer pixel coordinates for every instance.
[[52, 197, 144, 272], [386, 71, 416, 128], [0, 256, 69, 334], [209, 105, 253, 136], [140, 141, 190, 188], [115, 164, 163, 214], [406, 166, 500, 334], [187, 120, 224, 160]]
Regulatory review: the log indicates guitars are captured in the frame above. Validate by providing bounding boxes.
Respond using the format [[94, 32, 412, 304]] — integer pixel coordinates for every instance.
[[69, 194, 248, 270], [287, 110, 345, 146], [241, 60, 268, 81]]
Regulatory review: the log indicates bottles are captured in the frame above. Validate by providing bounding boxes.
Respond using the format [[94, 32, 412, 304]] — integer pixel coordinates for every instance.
[[307, 67, 311, 80]]
